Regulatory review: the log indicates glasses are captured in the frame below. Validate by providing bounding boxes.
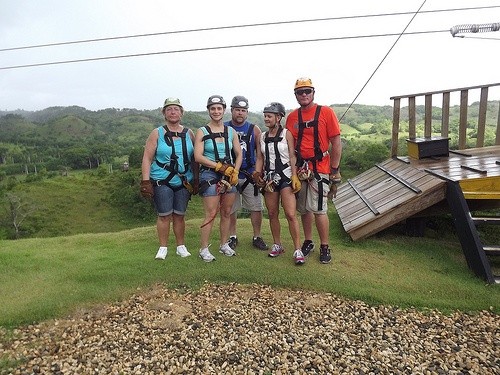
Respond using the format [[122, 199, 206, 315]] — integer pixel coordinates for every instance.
[[295, 89, 314, 95]]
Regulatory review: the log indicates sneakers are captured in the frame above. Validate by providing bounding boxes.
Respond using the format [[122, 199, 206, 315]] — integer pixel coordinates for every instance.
[[293, 249, 305, 264], [227, 237, 237, 250], [199, 244, 216, 262], [219, 244, 236, 256], [155, 246, 167, 260], [269, 243, 285, 257], [318, 246, 331, 264], [176, 245, 192, 258], [300, 241, 314, 255], [252, 236, 268, 250]]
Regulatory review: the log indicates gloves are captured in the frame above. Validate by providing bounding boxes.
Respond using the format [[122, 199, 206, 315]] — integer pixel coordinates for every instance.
[[251, 171, 264, 188], [290, 176, 302, 194], [329, 166, 342, 183], [215, 162, 233, 177], [140, 180, 153, 197], [230, 170, 239, 186]]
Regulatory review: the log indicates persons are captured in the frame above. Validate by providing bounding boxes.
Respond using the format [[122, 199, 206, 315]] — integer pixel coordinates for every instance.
[[141, 97, 199, 259], [252, 102, 306, 265], [286, 77, 341, 263], [223, 96, 270, 250], [193, 95, 242, 262]]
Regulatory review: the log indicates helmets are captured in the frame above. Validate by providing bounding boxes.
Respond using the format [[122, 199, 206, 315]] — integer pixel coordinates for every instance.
[[294, 78, 314, 90], [263, 103, 285, 118], [207, 96, 226, 109], [163, 98, 183, 111], [231, 96, 248, 109]]
